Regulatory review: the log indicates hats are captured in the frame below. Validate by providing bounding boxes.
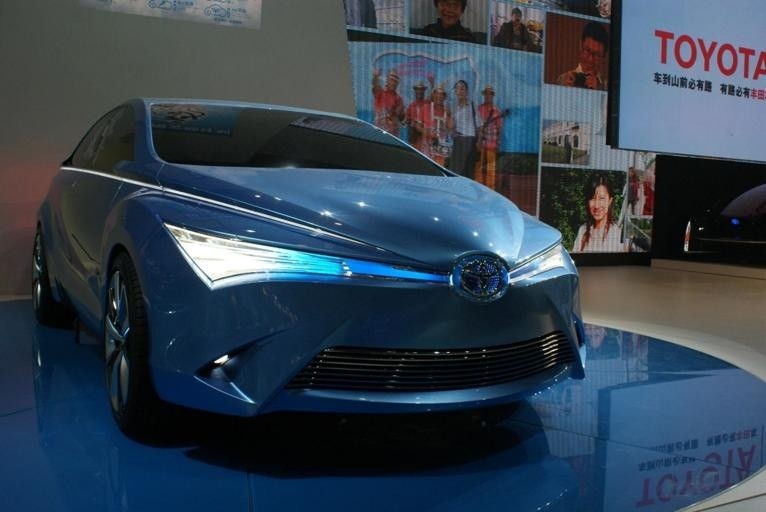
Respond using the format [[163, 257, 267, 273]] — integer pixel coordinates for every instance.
[[386, 71, 400, 83], [413, 81, 428, 90], [432, 85, 448, 96]]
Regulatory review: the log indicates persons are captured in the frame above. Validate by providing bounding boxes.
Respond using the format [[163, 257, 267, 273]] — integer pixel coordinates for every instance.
[[370, 66, 407, 138], [570, 170, 632, 253], [474, 84, 504, 190], [563, 123, 590, 164], [555, 20, 609, 91], [407, 79, 431, 146], [618, 166, 640, 215], [493, 7, 541, 54], [449, 80, 485, 180], [633, 154, 656, 215], [414, 0, 477, 43], [415, 84, 455, 167]]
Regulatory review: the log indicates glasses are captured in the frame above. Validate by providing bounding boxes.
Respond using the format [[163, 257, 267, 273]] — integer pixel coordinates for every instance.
[[580, 45, 605, 62], [482, 91, 495, 95]]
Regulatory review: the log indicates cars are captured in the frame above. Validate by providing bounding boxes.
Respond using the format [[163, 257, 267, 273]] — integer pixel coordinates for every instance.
[[27, 97, 588, 436]]
